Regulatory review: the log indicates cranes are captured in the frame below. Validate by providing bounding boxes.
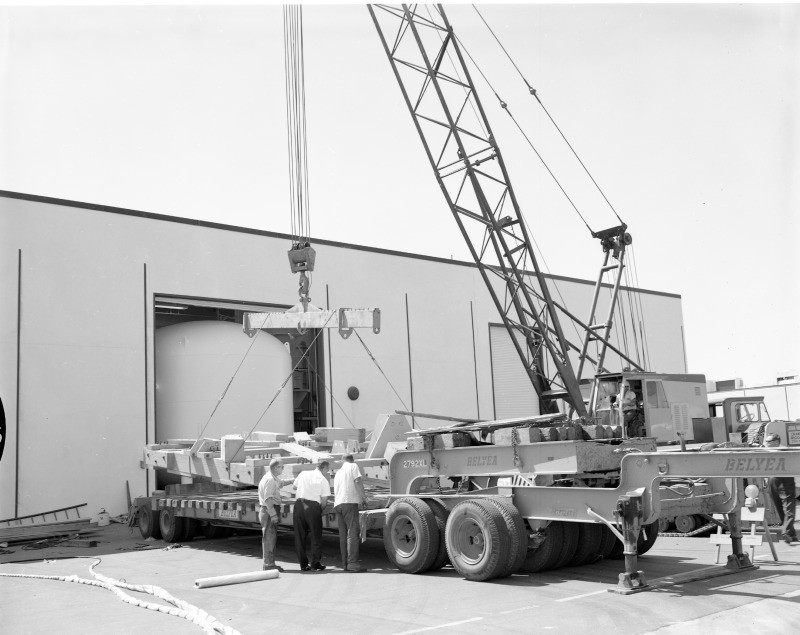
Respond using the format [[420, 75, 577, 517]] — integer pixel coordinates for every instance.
[[368, 2, 634, 432]]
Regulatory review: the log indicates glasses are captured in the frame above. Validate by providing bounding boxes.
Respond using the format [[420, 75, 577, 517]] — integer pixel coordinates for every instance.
[[275, 466, 284, 470]]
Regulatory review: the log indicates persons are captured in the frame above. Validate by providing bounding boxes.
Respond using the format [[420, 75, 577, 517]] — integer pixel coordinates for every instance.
[[765, 432, 799, 544], [333, 454, 368, 572], [292, 460, 332, 573], [258, 458, 296, 572], [613, 382, 637, 439]]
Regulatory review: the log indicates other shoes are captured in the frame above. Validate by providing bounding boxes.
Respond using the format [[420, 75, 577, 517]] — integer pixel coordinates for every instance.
[[343, 564, 347, 571], [301, 566, 311, 571], [263, 566, 283, 572], [347, 564, 367, 573], [310, 563, 325, 571]]
